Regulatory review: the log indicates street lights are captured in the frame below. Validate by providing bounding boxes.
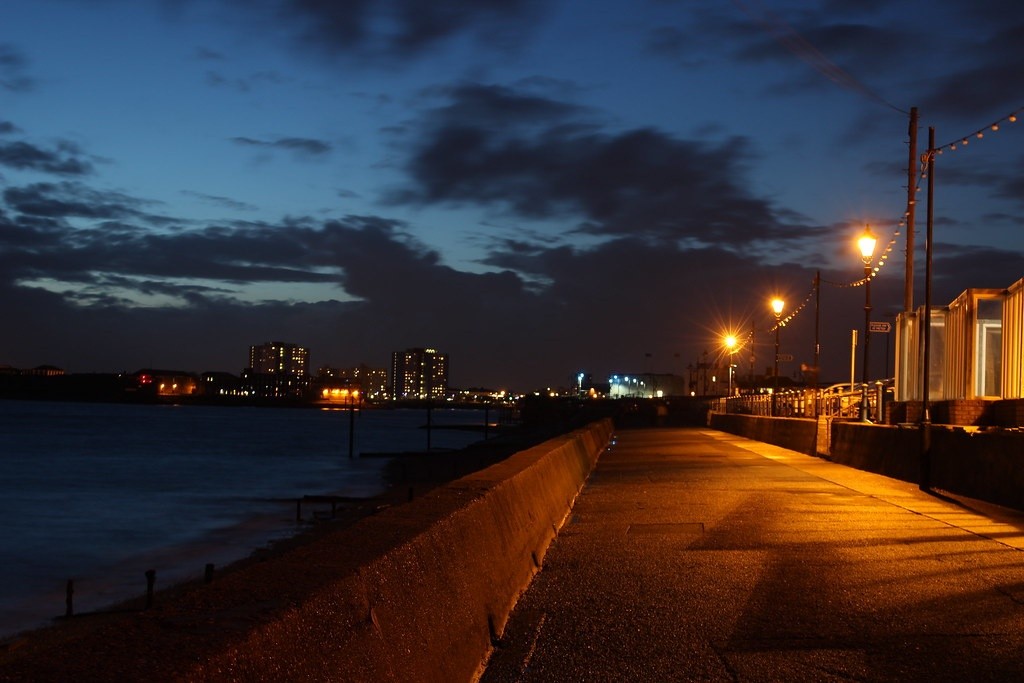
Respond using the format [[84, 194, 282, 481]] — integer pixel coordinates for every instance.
[[858, 223, 877, 424], [728, 336, 736, 397], [771, 298, 784, 417]]
